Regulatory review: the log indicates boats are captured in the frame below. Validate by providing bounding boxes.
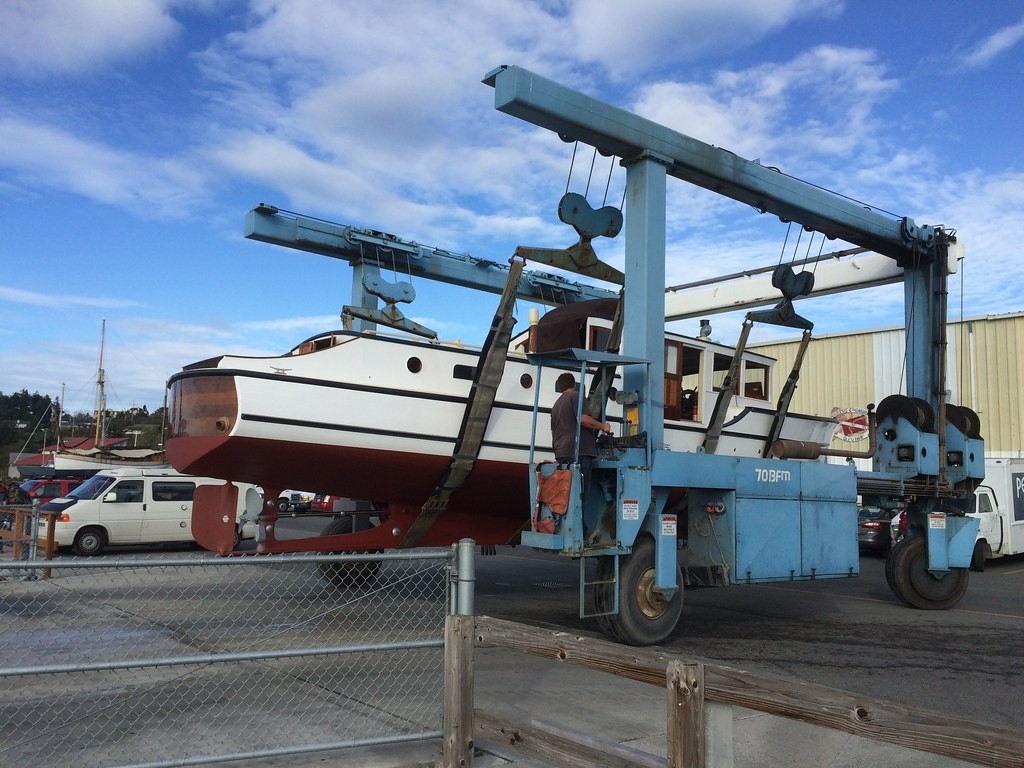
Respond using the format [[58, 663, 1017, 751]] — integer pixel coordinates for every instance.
[[162, 326, 840, 563]]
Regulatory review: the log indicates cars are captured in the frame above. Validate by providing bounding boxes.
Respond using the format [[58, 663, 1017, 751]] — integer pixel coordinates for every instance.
[[310, 494, 342, 512], [17, 478, 88, 506]]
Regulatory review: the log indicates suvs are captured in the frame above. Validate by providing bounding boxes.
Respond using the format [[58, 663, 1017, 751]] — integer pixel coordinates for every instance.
[[855, 505, 894, 559]]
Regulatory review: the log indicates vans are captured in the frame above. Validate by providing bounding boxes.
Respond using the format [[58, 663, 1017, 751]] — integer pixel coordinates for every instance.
[[36, 465, 263, 560]]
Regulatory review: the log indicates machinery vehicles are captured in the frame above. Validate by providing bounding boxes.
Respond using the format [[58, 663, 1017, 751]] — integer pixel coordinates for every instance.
[[240, 65, 986, 650]]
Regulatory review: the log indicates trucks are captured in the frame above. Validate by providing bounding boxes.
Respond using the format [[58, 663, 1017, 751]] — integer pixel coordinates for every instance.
[[886, 452, 1024, 570]]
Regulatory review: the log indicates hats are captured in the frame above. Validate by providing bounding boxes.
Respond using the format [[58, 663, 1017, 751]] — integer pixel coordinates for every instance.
[[558, 373, 576, 391]]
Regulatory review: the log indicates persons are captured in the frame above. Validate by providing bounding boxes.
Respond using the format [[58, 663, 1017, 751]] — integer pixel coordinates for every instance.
[[0, 481, 10, 554], [550, 373, 612, 546], [2, 475, 33, 561]]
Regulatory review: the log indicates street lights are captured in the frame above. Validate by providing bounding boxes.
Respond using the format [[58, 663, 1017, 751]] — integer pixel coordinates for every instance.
[[41, 428, 50, 466]]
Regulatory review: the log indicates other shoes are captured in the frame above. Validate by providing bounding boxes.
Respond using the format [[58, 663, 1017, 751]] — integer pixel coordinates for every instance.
[[592, 536, 614, 550]]
